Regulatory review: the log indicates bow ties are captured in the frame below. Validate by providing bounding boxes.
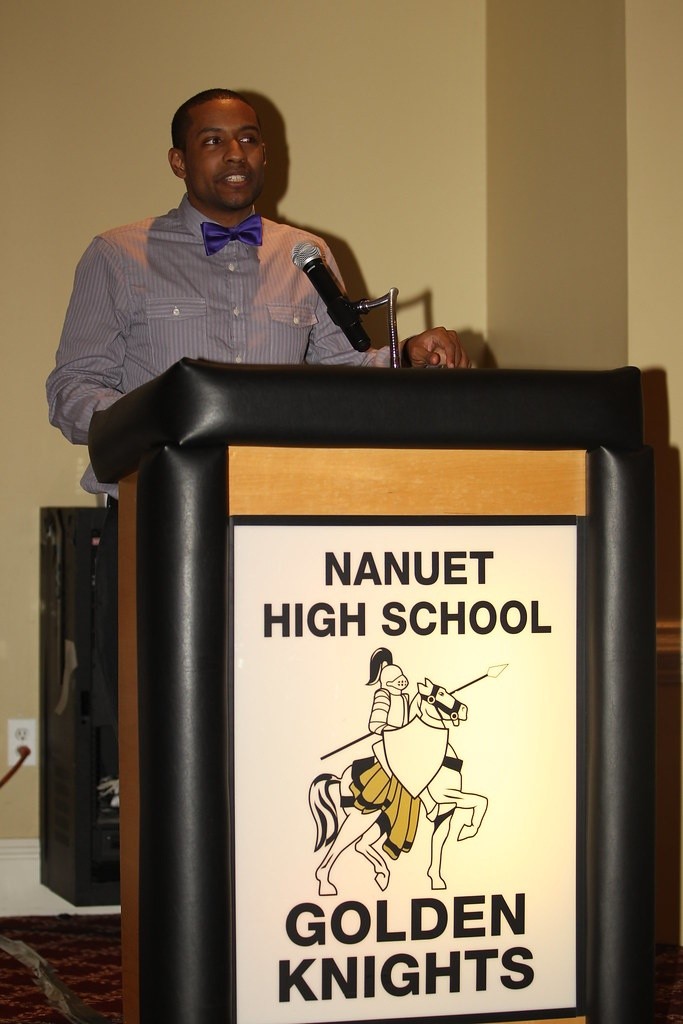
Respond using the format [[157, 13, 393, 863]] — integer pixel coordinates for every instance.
[[200, 214, 263, 256]]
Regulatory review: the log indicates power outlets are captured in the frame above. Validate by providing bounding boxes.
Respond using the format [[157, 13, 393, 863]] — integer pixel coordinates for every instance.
[[7, 718, 38, 767]]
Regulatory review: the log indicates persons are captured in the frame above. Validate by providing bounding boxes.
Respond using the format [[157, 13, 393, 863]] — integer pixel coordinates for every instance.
[[45, 88, 476, 779]]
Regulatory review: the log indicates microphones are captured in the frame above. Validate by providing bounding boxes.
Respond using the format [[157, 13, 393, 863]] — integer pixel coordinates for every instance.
[[292, 239, 372, 353]]
